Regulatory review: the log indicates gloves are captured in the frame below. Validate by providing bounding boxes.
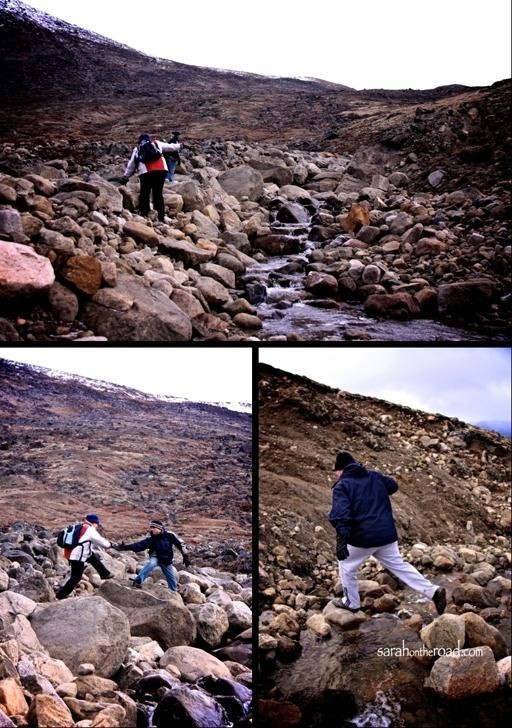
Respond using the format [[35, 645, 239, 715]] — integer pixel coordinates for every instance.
[[110, 541, 132, 551], [183, 556, 191, 568], [336, 536, 349, 561]]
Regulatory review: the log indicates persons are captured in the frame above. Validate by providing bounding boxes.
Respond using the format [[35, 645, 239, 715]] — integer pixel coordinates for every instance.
[[121, 134, 182, 224], [112, 518, 193, 594], [55, 512, 120, 601], [163, 130, 181, 184], [328, 450, 451, 616]]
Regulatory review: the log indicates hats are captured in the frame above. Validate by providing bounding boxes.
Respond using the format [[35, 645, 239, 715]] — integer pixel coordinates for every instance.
[[150, 520, 163, 530], [332, 452, 355, 472]]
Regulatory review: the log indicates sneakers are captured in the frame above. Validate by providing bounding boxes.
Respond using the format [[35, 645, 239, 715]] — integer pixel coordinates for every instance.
[[432, 587, 446, 615], [133, 581, 142, 588], [332, 598, 359, 613], [101, 574, 114, 579]]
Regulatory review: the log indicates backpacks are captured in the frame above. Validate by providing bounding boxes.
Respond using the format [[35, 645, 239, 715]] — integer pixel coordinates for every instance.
[[57, 523, 91, 549], [137, 139, 163, 163]]
